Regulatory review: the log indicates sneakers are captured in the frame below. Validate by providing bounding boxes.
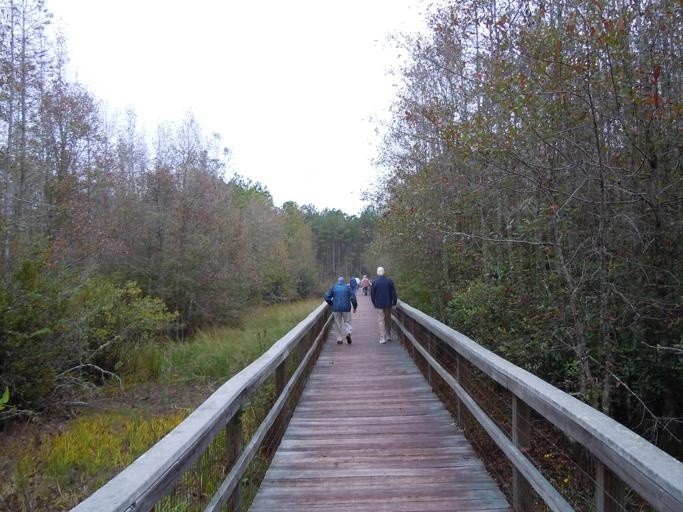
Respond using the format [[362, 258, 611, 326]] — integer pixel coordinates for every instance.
[[379, 335, 392, 343], [337, 334, 351, 344]]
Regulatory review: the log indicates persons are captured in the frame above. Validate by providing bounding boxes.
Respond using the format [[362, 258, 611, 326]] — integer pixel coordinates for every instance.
[[349, 275, 357, 293], [323, 276, 357, 345], [354, 276, 359, 290], [360, 274, 369, 296], [370, 266, 396, 344]]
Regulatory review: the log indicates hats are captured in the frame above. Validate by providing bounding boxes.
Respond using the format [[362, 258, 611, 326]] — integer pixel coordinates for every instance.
[[377, 267, 384, 276]]
